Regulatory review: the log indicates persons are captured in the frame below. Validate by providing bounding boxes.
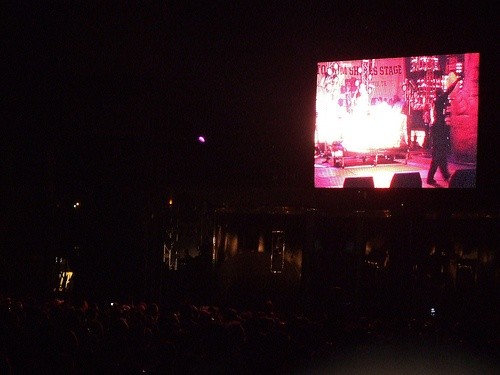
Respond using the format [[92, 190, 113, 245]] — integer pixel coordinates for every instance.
[[0, 293, 500, 375], [442, 240, 464, 290], [427, 76, 464, 185]]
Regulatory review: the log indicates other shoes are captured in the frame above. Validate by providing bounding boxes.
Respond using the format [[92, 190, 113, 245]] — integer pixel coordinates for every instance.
[[444, 174, 452, 180], [426, 178, 437, 184]]
